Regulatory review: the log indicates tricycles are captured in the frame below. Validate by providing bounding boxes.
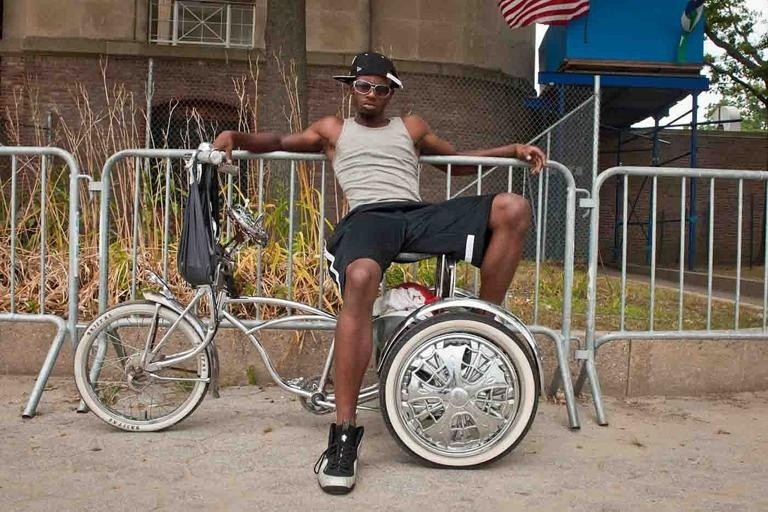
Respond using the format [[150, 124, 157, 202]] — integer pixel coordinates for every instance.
[[69, 137, 549, 472]]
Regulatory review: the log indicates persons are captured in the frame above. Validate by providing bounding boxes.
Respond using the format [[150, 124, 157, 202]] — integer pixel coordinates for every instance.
[[211, 51, 549, 498]]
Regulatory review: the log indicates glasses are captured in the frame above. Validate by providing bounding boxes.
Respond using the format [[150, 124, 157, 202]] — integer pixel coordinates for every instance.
[[353, 79, 392, 99]]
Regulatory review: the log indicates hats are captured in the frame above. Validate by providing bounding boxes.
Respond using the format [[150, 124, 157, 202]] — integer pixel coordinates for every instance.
[[332, 53, 403, 88]]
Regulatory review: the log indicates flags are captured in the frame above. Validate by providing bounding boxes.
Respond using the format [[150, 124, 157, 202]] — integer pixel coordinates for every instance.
[[497, 0, 593, 34]]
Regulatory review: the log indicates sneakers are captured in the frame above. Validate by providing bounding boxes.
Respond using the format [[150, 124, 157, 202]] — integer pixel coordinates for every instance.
[[313, 420, 364, 494]]
